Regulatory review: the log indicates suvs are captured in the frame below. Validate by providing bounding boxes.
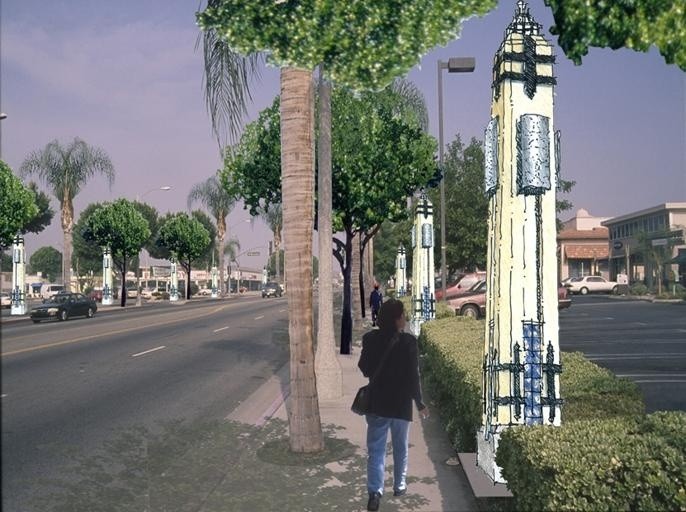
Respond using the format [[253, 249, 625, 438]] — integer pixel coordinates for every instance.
[[260, 281, 283, 299]]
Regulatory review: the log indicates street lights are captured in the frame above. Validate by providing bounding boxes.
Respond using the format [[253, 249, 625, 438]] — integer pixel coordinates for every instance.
[[135, 185, 174, 204], [225, 216, 252, 231], [0, 110, 8, 123], [436, 56, 477, 309]]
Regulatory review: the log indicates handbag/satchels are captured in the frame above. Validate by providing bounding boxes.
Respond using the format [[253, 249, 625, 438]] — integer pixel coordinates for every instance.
[[350, 384, 372, 416]]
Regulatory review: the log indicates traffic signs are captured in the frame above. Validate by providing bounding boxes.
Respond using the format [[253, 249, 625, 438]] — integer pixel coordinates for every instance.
[[245, 252, 261, 257]]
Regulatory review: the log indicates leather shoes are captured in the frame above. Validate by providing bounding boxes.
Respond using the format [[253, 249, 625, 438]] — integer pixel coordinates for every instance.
[[367, 492, 379, 511]]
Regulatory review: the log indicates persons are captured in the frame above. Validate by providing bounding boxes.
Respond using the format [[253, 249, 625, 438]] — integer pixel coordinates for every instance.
[[370, 283, 384, 327], [350, 298, 430, 511]]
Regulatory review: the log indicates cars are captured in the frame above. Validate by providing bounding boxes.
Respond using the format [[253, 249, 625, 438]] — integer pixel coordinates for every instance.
[[560, 276, 577, 287], [434, 271, 573, 325], [126, 286, 168, 299], [566, 275, 619, 295], [0, 290, 13, 308], [85, 289, 102, 302], [30, 292, 98, 323], [197, 285, 248, 296]]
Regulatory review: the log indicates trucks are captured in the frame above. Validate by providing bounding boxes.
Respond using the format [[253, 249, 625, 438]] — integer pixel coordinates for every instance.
[[40, 284, 64, 303]]
